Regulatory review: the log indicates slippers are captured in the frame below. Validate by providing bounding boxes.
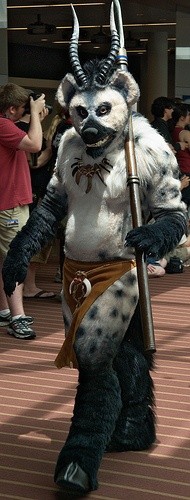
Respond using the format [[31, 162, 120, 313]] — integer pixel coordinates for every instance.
[[23, 289, 56, 299]]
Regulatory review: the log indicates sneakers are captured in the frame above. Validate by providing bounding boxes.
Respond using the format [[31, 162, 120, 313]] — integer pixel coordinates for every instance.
[[7, 316, 36, 339], [0, 310, 34, 326]]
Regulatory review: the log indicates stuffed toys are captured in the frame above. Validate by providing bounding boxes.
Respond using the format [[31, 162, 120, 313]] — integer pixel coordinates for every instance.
[[2, 2, 186, 497]]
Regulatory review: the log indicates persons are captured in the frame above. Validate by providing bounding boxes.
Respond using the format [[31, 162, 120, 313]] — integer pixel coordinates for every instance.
[[0, 80, 190, 339]]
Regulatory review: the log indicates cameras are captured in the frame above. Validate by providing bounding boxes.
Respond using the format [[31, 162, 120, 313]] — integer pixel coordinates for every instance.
[[26, 92, 53, 115]]
